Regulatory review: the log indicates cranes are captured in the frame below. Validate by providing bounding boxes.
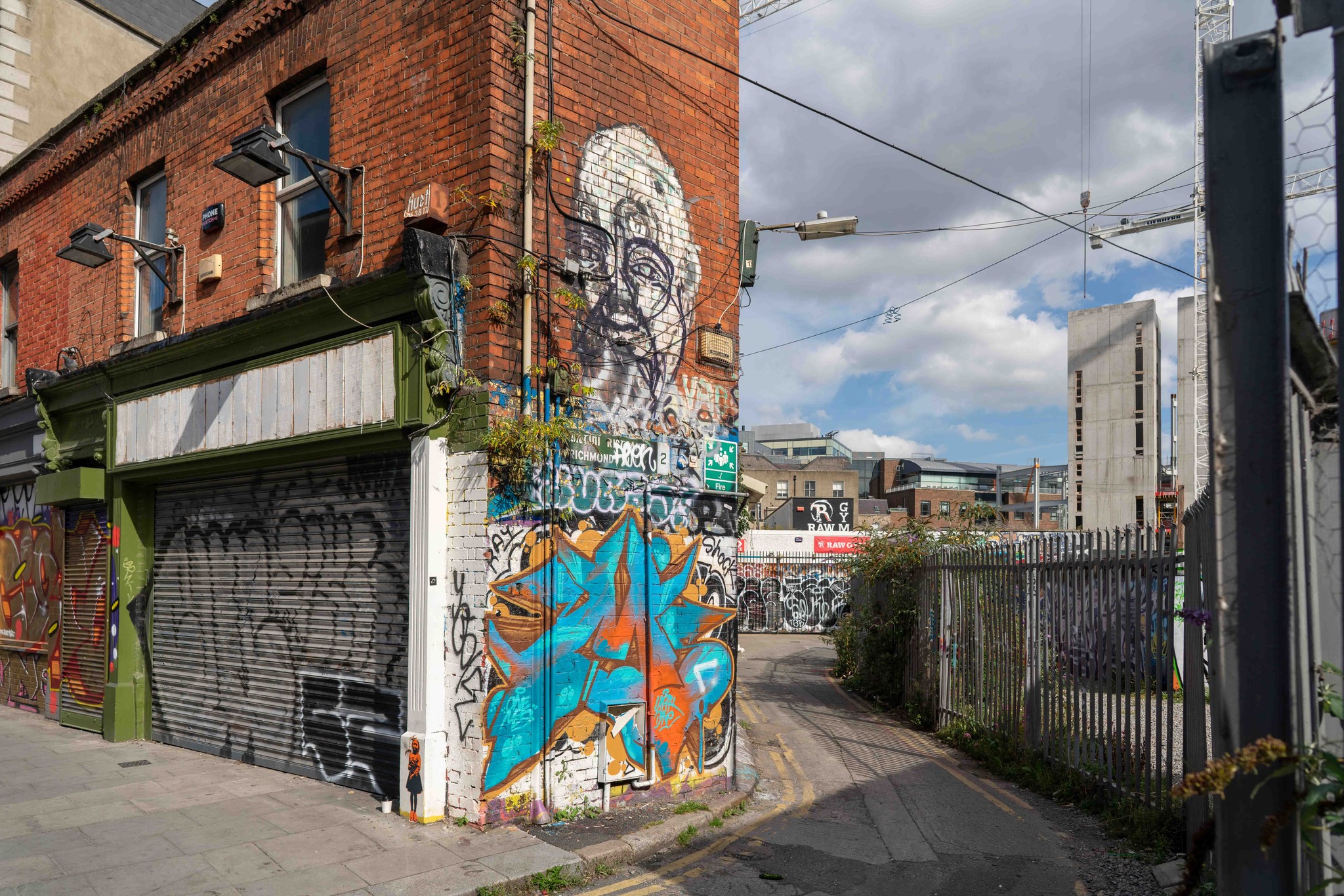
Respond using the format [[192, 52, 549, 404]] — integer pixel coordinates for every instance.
[[1079, 0, 1337, 503]]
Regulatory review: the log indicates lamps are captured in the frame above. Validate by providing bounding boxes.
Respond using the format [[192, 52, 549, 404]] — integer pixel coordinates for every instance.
[[757, 210, 858, 241], [56, 222, 183, 307], [213, 124, 363, 242]]
